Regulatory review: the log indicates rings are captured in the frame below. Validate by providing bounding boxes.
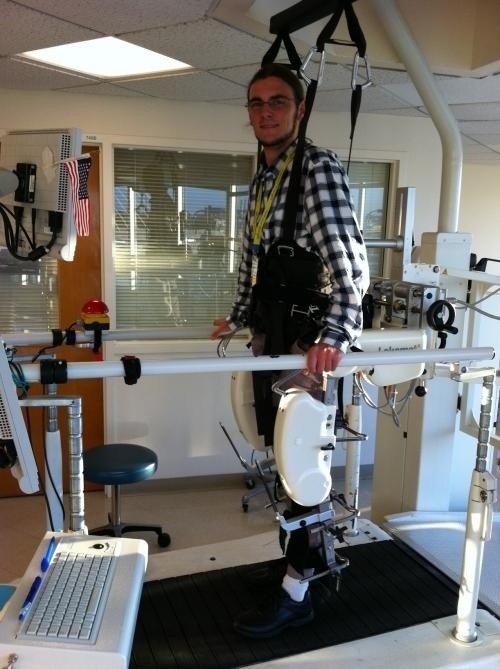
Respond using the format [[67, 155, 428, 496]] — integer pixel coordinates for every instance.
[[17, 576, 43, 621], [40, 536, 57, 572]]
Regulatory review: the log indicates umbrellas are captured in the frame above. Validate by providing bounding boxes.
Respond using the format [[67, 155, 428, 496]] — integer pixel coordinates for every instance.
[[231, 584, 315, 639]]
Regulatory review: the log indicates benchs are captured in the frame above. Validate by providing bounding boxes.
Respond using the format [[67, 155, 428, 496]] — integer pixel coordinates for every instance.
[[60, 153, 92, 237]]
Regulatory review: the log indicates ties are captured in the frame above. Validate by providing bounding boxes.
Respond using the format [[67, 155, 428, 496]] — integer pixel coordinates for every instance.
[[0, 127, 82, 262]]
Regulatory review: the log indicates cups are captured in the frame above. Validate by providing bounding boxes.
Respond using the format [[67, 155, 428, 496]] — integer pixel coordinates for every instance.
[[245, 97, 297, 109]]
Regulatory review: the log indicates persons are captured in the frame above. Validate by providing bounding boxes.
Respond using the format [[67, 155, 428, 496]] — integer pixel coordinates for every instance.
[[206, 65, 375, 639], [141, 168, 186, 326]]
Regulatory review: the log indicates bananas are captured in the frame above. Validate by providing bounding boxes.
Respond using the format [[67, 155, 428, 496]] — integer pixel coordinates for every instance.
[[26, 551, 118, 646]]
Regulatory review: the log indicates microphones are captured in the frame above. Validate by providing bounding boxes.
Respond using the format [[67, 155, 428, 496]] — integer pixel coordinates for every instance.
[[80, 443, 173, 550]]
